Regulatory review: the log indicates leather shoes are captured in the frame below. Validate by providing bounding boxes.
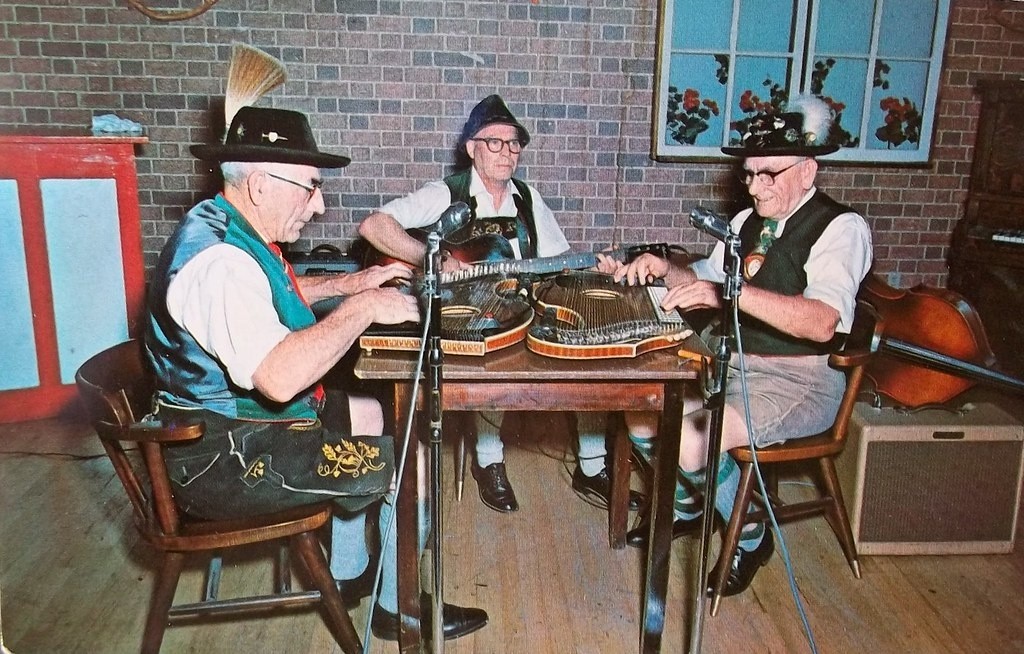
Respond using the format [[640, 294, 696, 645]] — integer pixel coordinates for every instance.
[[707, 524, 774, 597], [470, 451, 520, 514], [332, 554, 383, 611], [573, 461, 647, 511], [371, 587, 490, 642], [625, 506, 721, 548]]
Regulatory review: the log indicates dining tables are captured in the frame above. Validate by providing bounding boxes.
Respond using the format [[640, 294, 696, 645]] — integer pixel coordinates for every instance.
[[354, 263, 737, 654]]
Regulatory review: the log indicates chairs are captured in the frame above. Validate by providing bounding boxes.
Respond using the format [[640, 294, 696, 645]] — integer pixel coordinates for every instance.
[[74, 337, 367, 654], [710, 297, 890, 616]]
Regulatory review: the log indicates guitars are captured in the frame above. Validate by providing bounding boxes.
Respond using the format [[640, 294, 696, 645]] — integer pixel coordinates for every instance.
[[363, 229, 671, 288]]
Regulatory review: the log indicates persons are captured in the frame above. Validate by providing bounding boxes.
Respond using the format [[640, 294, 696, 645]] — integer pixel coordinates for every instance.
[[356, 91, 644, 515], [611, 111, 877, 598], [141, 110, 489, 642]]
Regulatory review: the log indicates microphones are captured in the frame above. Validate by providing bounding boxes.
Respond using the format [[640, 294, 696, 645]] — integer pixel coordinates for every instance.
[[427, 200, 471, 244], [688, 206, 742, 250]]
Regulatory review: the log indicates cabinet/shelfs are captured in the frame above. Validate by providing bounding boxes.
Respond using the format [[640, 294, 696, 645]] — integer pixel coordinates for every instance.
[[0, 127, 152, 425]]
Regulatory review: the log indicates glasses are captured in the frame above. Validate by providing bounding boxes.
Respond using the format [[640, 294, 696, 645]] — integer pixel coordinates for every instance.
[[736, 160, 806, 186], [267, 173, 325, 203]]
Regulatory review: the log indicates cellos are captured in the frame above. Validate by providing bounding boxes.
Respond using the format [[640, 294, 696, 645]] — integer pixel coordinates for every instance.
[[849, 269, 1024, 415]]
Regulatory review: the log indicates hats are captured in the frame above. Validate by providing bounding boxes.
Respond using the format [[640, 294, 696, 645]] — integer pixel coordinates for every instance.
[[721, 96, 840, 157], [188, 105, 352, 169], [458, 94, 530, 160], [469, 137, 522, 154]]
